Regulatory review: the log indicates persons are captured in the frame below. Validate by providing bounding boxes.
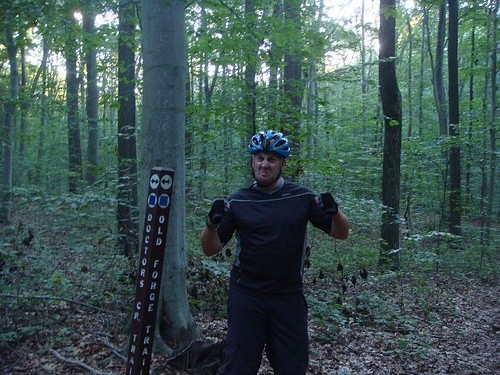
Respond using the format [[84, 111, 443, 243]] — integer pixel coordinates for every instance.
[[200, 130, 349, 375]]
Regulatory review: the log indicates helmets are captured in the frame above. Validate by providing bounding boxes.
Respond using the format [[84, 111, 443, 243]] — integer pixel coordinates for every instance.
[[247, 130, 291, 157]]
